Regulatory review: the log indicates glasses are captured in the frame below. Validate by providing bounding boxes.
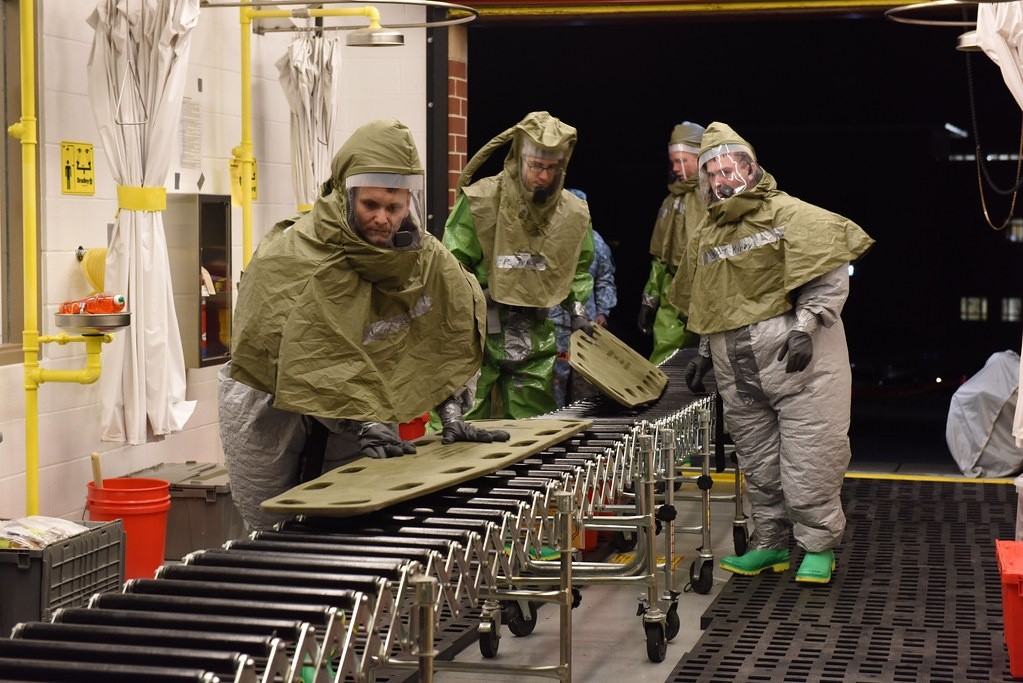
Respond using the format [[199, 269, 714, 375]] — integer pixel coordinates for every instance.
[[524, 159, 562, 175]]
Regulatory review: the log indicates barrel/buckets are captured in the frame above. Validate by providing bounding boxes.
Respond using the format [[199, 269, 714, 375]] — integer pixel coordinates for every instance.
[[82, 478, 171, 581]]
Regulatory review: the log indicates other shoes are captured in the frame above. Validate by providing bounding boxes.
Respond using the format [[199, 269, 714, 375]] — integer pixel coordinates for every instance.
[[297, 645, 342, 682], [794, 549, 837, 584], [501, 528, 561, 562], [718, 547, 791, 576]]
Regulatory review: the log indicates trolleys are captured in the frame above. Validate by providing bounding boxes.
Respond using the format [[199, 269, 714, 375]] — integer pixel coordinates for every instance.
[[0, 347, 749, 683]]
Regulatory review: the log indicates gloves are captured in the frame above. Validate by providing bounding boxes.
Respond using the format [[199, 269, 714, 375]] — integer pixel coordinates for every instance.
[[637, 304, 656, 334], [438, 402, 511, 444], [345, 417, 417, 459], [569, 317, 593, 338], [684, 354, 713, 393], [482, 340, 490, 367], [776, 330, 813, 374]]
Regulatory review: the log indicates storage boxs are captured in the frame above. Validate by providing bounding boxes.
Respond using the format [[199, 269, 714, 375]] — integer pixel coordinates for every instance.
[[0, 518, 128, 639], [119, 462, 249, 561], [995, 538, 1023, 678]]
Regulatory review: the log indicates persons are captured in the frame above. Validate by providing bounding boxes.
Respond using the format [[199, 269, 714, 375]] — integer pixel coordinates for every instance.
[[666, 122, 875, 583], [637, 121, 705, 367], [218, 118, 509, 533], [426, 110, 594, 560], [546, 190, 616, 410]]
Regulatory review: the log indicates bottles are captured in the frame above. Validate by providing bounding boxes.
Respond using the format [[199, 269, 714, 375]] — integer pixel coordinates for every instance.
[[61, 293, 125, 317]]
